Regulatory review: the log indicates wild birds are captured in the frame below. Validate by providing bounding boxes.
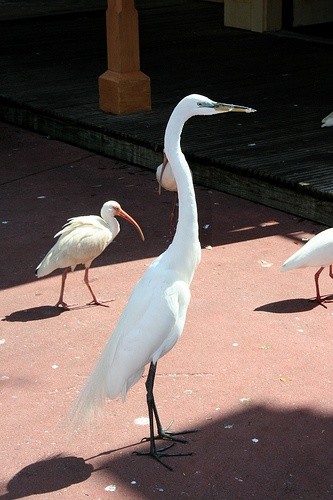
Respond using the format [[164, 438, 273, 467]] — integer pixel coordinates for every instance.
[[32, 199, 145, 309], [154, 149, 180, 245], [278, 226, 333, 309], [74, 93, 257, 474]]
